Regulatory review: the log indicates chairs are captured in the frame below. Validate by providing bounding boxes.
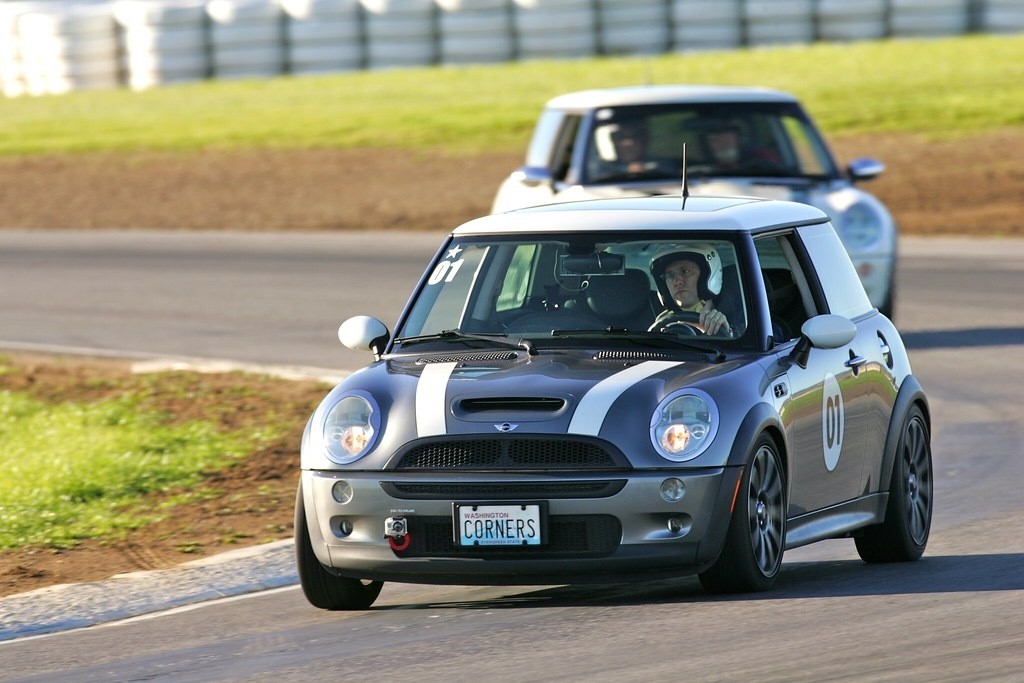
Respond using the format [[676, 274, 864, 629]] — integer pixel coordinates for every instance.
[[559, 270, 651, 334], [714, 265, 790, 345]]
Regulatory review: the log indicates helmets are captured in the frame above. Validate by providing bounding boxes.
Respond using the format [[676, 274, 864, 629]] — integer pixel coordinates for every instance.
[[594, 124, 650, 161], [697, 119, 753, 161], [649, 242, 722, 306]]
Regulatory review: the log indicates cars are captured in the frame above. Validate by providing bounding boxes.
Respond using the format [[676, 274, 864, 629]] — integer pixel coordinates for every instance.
[[291, 193, 935, 617], [488, 82, 899, 321]]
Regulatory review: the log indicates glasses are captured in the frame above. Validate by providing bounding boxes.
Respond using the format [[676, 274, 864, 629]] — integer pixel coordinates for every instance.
[[662, 265, 699, 281]]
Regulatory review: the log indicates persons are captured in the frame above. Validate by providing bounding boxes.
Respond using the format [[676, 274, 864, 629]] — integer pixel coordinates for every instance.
[[594, 120, 675, 178], [647, 242, 784, 345], [686, 119, 776, 175]]
